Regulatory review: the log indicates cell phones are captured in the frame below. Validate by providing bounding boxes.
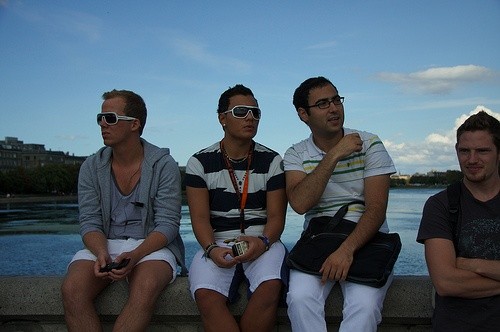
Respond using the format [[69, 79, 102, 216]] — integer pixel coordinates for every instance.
[[232, 241, 248, 256], [100, 259, 130, 273]]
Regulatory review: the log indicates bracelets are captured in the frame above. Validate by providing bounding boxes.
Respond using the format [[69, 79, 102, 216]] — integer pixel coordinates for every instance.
[[258, 235, 270, 251], [201, 242, 219, 262]]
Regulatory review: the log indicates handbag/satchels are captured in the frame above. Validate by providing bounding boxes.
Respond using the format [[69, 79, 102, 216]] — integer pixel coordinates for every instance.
[[287, 199, 403, 289]]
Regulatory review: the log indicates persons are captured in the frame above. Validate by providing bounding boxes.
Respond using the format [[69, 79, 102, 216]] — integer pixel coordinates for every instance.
[[416, 110, 500, 332], [185, 85, 287, 332], [283, 76, 397, 332], [62, 89, 189, 332]]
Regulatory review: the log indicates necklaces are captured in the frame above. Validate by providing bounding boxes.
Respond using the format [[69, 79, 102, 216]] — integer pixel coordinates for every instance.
[[222, 142, 250, 193]]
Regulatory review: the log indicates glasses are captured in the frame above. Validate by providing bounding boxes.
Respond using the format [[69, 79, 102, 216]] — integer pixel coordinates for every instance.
[[97, 112, 137, 126], [223, 105, 261, 120], [305, 97, 345, 109]]
